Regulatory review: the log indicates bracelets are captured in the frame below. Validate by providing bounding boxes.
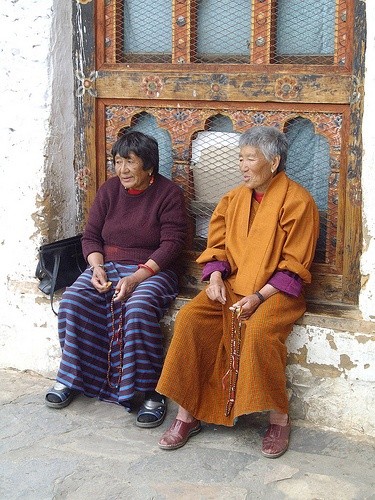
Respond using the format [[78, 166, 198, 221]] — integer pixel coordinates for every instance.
[[89, 263, 104, 269], [255, 291, 264, 302], [137, 263, 155, 276]]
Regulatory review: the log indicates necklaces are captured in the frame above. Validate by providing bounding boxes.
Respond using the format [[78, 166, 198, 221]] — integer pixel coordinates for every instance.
[[224, 304, 241, 417], [106, 291, 124, 389]]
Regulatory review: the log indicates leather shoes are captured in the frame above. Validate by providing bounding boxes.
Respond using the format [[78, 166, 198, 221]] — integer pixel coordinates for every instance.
[[260, 413, 292, 459], [157, 418, 202, 450]]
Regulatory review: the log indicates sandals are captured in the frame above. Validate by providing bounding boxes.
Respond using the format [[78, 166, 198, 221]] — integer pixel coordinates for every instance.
[[135, 399, 168, 428], [45, 381, 77, 409]]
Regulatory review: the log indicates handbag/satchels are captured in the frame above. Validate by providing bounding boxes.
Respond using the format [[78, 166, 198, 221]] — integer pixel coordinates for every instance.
[[35, 233, 89, 317]]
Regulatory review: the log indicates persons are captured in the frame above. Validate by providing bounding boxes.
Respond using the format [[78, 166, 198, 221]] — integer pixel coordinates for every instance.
[[159, 123, 320, 459], [43, 130, 197, 429]]
